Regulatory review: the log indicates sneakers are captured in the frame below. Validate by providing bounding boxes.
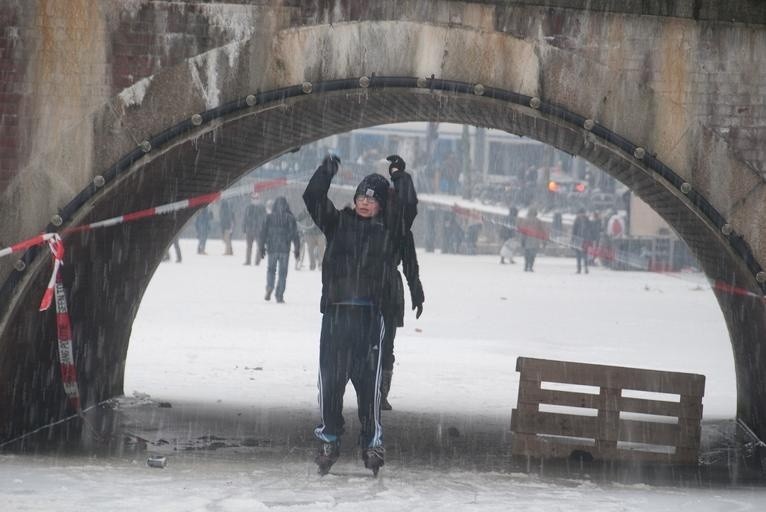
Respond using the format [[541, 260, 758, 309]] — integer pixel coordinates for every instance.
[[363, 447, 384, 468], [381, 399, 392, 410], [315, 442, 340, 468]]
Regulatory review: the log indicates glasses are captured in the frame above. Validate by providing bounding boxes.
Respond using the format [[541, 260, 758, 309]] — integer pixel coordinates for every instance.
[[355, 193, 377, 203]]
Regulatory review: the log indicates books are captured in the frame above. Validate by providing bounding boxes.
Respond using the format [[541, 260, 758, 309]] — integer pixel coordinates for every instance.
[[164, 195, 267, 267]]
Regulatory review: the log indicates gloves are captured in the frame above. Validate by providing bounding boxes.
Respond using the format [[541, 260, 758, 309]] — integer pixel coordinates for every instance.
[[386, 155, 405, 176], [322, 155, 341, 177], [412, 303, 423, 319]]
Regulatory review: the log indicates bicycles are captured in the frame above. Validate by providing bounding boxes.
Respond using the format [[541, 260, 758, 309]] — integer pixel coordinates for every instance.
[[293, 221, 313, 271]]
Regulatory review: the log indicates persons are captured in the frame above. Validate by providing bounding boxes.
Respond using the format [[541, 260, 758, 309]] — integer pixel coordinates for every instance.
[[258, 194, 302, 304], [303, 153, 418, 471], [412, 149, 463, 195], [303, 203, 327, 273], [358, 148, 391, 178], [427, 207, 619, 274], [515, 161, 538, 208], [379, 187, 425, 411]]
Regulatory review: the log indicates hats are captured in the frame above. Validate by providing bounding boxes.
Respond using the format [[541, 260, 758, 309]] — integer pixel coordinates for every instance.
[[354, 173, 389, 208]]
